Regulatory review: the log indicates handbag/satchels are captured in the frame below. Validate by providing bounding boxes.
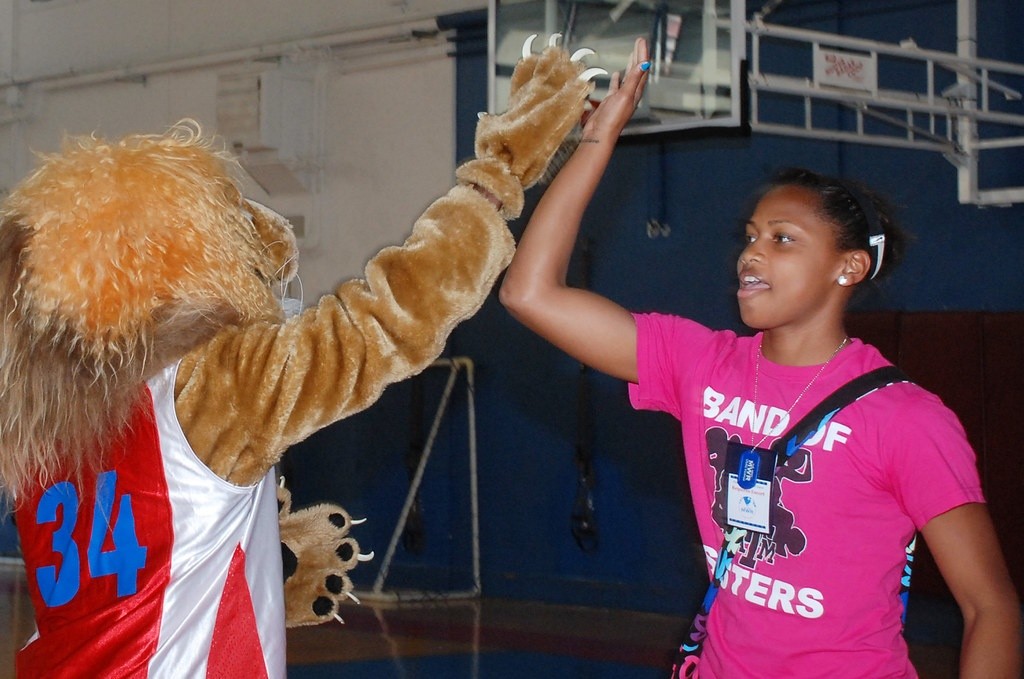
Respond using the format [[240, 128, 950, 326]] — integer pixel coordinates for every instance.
[[670, 642, 702, 679]]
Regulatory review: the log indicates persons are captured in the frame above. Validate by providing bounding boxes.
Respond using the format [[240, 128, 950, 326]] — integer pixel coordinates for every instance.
[[0, 33, 609, 679], [500, 37, 1021, 679]]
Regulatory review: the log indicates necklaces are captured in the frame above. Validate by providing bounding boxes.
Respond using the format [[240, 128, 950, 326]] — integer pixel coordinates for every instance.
[[737, 332, 850, 489]]
